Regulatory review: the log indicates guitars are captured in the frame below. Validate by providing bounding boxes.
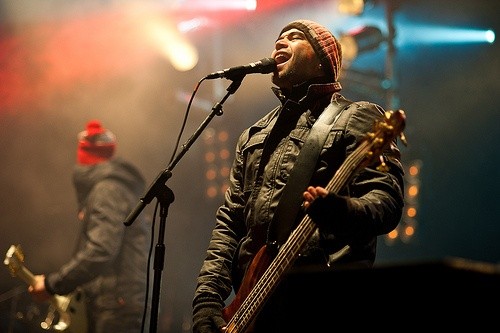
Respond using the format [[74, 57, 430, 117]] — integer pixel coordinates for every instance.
[[3, 243, 89, 333], [221, 108, 407, 333]]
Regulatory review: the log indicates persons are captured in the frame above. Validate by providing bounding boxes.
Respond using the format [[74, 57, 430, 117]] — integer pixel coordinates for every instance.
[[28, 119, 156, 333], [191, 19, 404, 333]]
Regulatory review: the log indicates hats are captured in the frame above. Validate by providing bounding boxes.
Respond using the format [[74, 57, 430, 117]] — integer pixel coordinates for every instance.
[[77, 121, 117, 166], [277, 20, 343, 81]]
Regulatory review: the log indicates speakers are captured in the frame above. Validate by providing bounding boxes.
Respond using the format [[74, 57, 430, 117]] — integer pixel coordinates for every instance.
[[372, 258, 500, 333]]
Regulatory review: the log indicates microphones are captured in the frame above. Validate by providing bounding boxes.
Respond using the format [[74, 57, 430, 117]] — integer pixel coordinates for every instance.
[[207, 57, 276, 80]]
